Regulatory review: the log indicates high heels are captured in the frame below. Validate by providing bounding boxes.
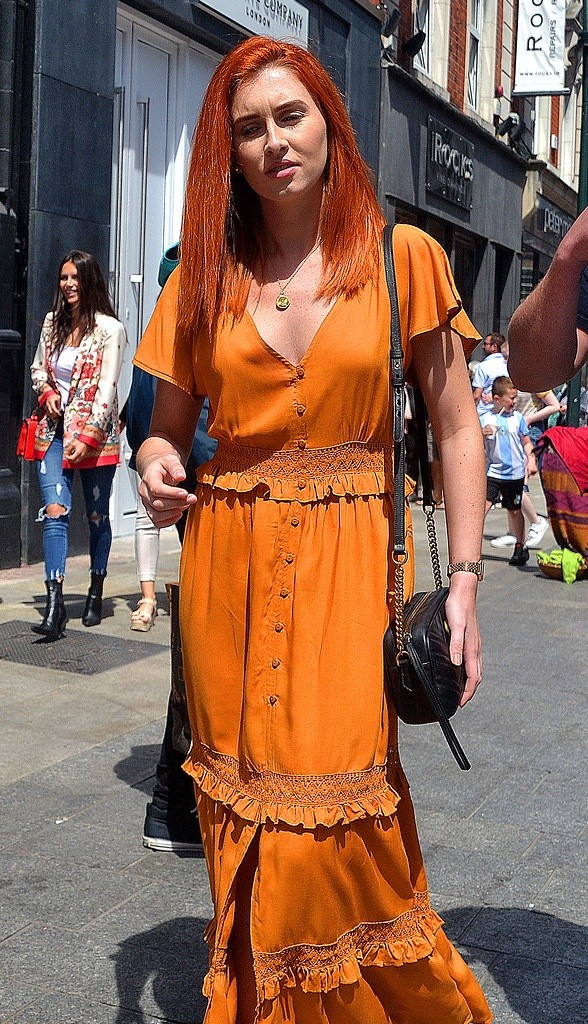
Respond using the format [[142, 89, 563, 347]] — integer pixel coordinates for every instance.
[[129, 597, 157, 631]]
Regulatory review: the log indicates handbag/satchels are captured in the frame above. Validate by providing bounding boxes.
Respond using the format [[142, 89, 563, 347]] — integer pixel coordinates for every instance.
[[385, 586, 472, 773], [15, 391, 59, 462]]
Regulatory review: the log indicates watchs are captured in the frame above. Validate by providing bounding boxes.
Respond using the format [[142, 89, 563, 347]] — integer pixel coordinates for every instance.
[[447, 562, 486, 581]]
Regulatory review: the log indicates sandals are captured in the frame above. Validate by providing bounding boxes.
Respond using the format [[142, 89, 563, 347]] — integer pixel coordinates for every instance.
[[434, 501, 445, 509]]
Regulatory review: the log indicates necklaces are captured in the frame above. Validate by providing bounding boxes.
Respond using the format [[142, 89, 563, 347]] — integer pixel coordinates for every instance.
[[262, 239, 322, 308]]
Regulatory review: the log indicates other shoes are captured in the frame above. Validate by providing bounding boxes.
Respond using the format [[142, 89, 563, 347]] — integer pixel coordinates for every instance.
[[523, 485, 530, 496], [408, 492, 424, 502]]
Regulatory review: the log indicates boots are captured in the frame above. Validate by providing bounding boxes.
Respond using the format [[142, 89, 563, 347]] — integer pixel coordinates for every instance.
[[30, 577, 69, 638], [82, 587, 102, 626]]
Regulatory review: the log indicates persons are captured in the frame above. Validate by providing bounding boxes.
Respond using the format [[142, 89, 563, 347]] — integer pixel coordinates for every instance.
[[536, 424, 588, 559], [121, 39, 493, 1024], [491, 342, 560, 549], [507, 207, 588, 392], [31, 247, 129, 641], [468, 329, 506, 408], [479, 377, 541, 565]]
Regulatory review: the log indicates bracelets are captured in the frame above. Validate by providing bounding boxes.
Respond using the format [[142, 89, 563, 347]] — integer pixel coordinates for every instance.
[[137, 452, 182, 473]]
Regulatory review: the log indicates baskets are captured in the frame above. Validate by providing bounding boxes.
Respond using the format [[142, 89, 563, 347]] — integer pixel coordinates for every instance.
[[536, 551, 588, 581]]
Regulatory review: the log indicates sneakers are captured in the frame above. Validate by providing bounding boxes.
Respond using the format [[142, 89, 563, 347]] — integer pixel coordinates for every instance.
[[142, 801, 205, 852], [491, 535, 517, 549], [508, 543, 530, 566], [525, 516, 549, 549]]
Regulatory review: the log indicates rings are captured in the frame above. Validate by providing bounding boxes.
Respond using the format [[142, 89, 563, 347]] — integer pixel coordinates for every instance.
[[76, 459, 80, 463]]
